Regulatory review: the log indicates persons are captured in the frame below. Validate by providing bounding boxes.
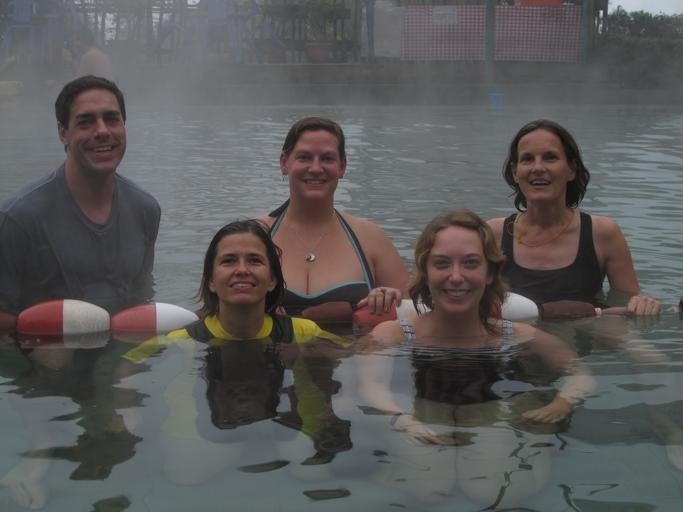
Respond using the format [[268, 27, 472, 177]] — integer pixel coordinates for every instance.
[[483, 118, 663, 318], [116, 221, 356, 369], [252, 116, 413, 326], [42, 27, 119, 85], [0, 74, 161, 335], [356, 207, 600, 447]]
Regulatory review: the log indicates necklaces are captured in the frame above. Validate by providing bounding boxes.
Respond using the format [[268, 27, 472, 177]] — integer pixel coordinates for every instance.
[[506, 209, 579, 248], [282, 208, 336, 262]]
